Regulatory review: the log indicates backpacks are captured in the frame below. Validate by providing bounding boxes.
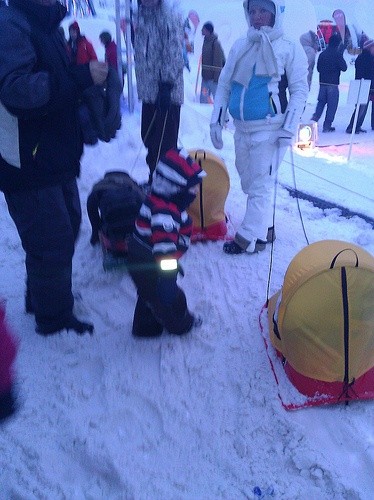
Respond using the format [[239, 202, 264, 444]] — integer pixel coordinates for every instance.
[[87, 171, 145, 254]]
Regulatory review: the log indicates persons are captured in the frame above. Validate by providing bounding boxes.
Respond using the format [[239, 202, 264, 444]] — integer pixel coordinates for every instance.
[[300, 30, 319, 92], [346, 39, 374, 134], [199, 21, 226, 104], [67, 21, 125, 97], [127, 146, 208, 338], [210, 0, 308, 255], [0, 0, 108, 336], [120, 0, 184, 187], [87, 171, 146, 247], [310, 34, 348, 132]]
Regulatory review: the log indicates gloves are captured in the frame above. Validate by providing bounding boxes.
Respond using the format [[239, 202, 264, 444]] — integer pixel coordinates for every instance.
[[156, 81, 174, 112], [86, 86, 116, 143], [210, 125, 223, 149], [270, 130, 291, 147], [105, 69, 123, 130], [158, 258, 184, 286]]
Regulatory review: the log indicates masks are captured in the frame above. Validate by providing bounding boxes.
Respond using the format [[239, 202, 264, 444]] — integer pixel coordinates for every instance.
[[178, 191, 197, 208]]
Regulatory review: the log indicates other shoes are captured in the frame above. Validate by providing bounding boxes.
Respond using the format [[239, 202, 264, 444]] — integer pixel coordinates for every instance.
[[323, 122, 334, 131], [24, 296, 35, 315], [356, 130, 367, 134], [310, 115, 319, 121], [257, 228, 277, 244], [223, 241, 265, 253], [192, 312, 202, 328], [346, 129, 351, 133], [36, 322, 93, 336]]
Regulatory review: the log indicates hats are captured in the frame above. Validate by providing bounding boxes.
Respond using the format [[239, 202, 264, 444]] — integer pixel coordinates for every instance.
[[248, 0, 274, 15], [363, 39, 374, 49]]
